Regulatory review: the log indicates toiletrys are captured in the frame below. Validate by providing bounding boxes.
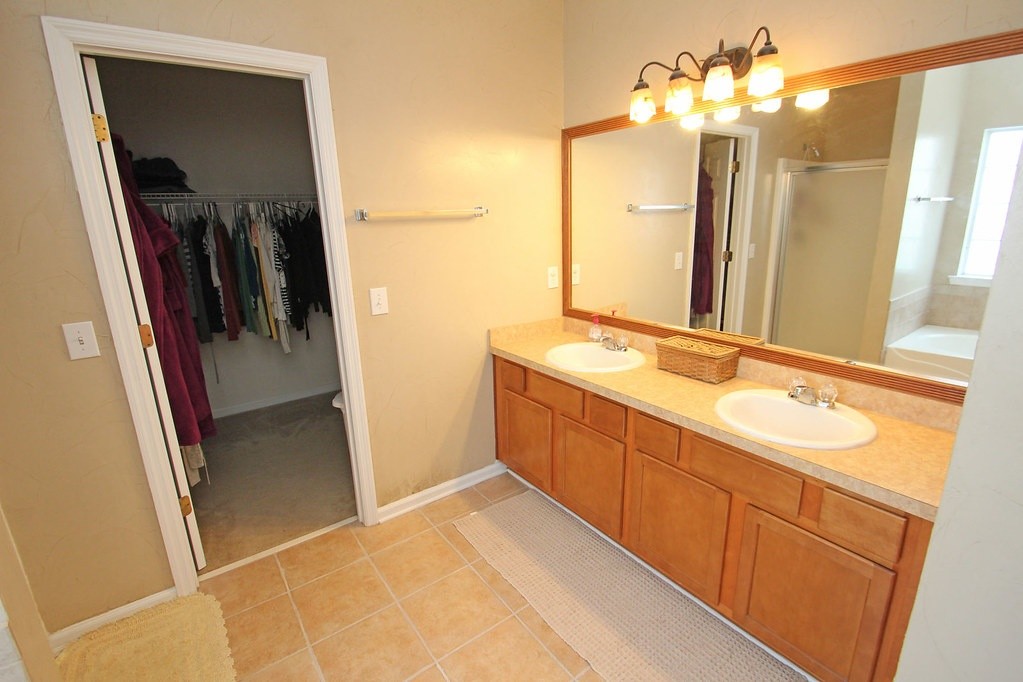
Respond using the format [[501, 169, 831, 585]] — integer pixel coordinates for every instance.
[[589, 313, 602, 342]]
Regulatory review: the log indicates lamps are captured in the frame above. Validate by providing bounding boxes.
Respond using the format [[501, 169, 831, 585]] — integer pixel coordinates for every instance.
[[629, 27, 785, 124], [680, 89, 830, 128]]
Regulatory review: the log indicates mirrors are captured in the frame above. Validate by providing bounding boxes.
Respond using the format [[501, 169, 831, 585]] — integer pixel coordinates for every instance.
[[560, 29, 1023, 404]]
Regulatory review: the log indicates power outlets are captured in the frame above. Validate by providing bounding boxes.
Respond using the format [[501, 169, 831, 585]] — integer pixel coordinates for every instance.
[[62, 321, 100, 361]]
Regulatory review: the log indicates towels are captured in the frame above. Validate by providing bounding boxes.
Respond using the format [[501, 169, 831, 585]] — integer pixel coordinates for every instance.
[[690, 163, 714, 314], [118, 175, 217, 445]]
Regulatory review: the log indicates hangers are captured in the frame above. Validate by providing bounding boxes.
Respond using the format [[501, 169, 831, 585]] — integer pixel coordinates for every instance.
[[159, 192, 313, 226]]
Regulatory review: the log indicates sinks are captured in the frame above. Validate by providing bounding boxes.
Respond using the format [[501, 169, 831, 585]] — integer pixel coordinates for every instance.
[[543, 342, 645, 373], [715, 390, 877, 449]]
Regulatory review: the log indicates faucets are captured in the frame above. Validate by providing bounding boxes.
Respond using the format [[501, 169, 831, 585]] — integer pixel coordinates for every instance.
[[599, 335, 627, 351], [787, 376, 838, 409]]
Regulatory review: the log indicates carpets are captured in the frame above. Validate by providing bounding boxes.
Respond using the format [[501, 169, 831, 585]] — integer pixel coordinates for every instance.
[[452, 491, 819, 682], [51, 592, 237, 682]]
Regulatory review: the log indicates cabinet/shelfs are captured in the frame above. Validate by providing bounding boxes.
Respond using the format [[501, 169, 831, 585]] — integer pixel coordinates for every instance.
[[490, 354, 932, 682]]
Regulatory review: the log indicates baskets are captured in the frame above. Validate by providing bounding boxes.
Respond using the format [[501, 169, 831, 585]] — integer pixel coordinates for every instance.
[[656, 334, 740, 385], [697, 328, 766, 346]]
